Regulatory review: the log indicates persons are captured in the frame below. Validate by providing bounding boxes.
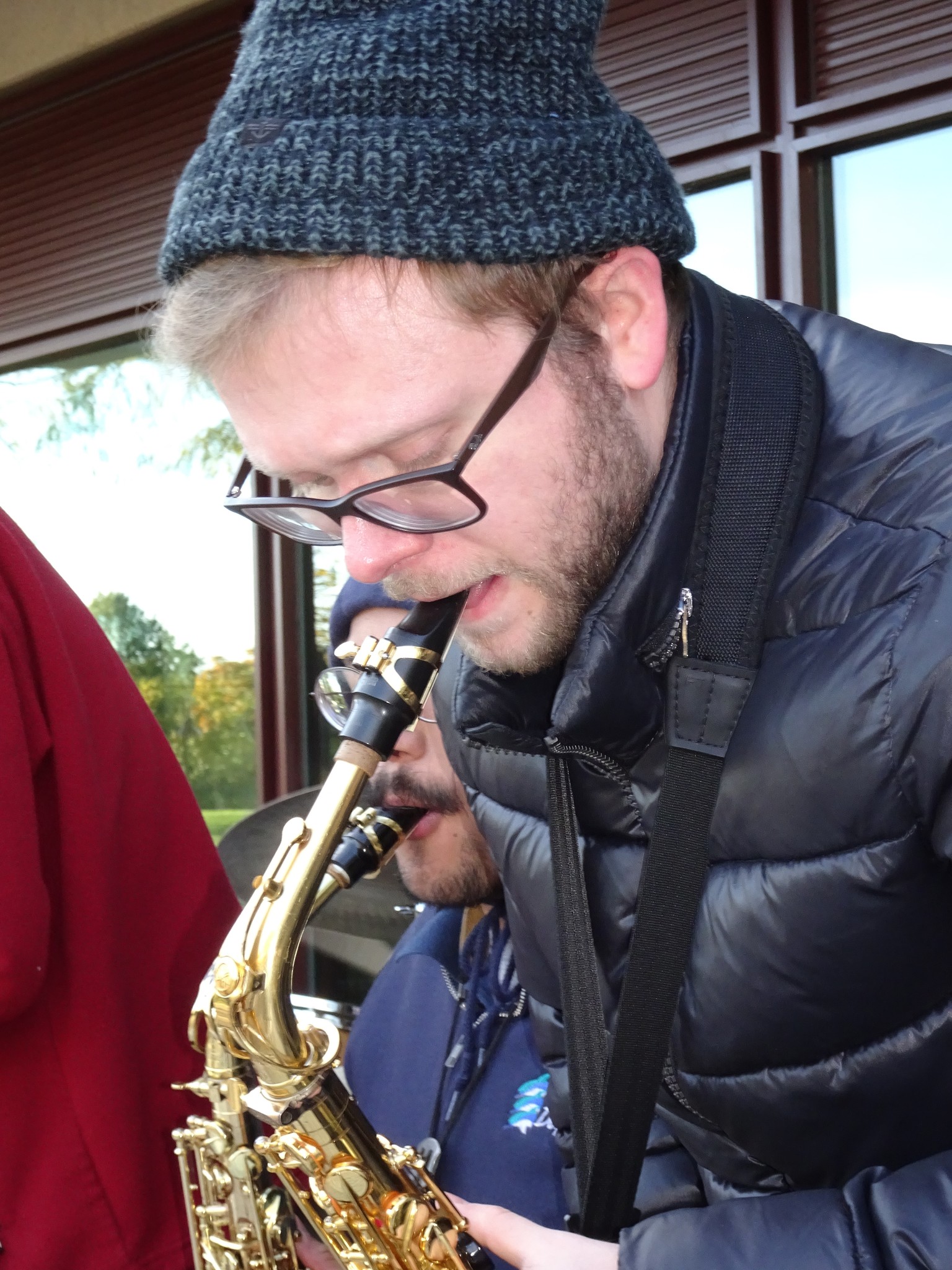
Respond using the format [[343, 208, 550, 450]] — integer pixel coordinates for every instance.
[[152, 1, 952, 1270], [0, 511, 242, 1270], [294, 570, 565, 1270]]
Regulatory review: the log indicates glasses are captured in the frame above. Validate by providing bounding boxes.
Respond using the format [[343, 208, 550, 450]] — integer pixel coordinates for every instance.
[[225, 259, 613, 547], [311, 668, 373, 736]]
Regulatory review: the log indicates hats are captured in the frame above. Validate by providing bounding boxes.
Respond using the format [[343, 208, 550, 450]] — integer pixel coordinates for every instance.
[[156, 0, 697, 281], [326, 572, 424, 717]]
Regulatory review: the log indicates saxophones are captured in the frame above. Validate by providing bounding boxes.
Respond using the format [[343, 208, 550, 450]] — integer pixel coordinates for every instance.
[[216, 593, 496, 1270], [173, 804, 420, 1270]]
